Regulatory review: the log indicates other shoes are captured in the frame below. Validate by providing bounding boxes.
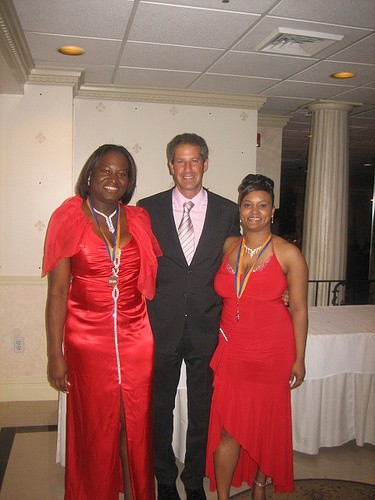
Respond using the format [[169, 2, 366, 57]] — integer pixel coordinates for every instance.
[[252, 477, 267, 500]]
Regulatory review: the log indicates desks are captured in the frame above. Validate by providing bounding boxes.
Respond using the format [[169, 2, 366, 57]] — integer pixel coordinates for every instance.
[[55, 305, 375, 469]]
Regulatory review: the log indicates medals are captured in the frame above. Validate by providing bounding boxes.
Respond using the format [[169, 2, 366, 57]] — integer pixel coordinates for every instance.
[[236, 308, 240, 322], [108, 275, 118, 286]]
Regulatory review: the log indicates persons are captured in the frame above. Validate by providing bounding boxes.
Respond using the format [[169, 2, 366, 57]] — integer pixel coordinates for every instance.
[[69, 133, 290, 500], [205, 173, 309, 500], [42, 144, 163, 500]]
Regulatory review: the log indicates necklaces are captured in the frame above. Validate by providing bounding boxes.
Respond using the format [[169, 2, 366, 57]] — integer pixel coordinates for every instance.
[[93, 207, 118, 234], [243, 244, 264, 256]]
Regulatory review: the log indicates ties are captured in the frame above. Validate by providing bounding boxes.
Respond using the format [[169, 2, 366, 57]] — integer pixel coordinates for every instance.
[[178, 201, 195, 266]]
